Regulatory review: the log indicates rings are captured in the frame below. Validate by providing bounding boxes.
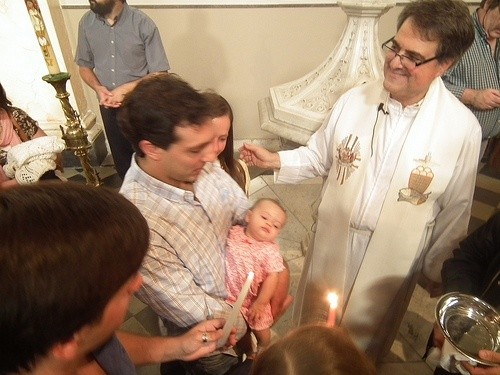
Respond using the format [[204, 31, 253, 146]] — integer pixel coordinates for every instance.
[[203, 333, 208, 342]]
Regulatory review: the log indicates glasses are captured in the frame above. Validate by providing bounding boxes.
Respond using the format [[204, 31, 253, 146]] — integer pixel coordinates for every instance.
[[381, 35, 438, 72]]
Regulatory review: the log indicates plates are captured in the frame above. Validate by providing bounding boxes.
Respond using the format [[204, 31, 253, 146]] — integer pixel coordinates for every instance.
[[435, 292, 500, 366]]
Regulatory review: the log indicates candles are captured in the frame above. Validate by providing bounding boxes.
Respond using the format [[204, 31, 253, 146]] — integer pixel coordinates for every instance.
[[214, 270, 254, 348], [22, 0, 60, 74], [327, 288, 339, 328]]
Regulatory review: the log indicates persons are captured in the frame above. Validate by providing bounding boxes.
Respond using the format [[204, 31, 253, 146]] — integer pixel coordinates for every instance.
[[0, 82, 65, 187], [439, 0, 500, 175], [196, 90, 250, 197], [115, 71, 292, 375], [74, 0, 171, 183], [238, 0, 483, 365], [434, 209, 500, 375], [249, 324, 375, 375], [0, 182, 150, 375], [224, 197, 286, 348]]
